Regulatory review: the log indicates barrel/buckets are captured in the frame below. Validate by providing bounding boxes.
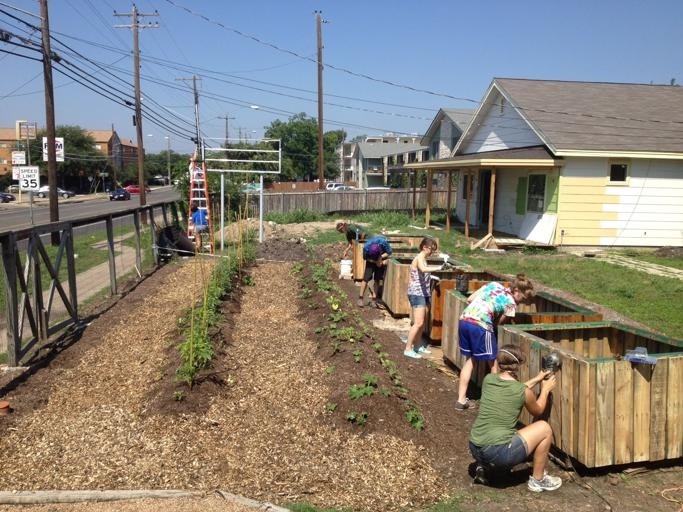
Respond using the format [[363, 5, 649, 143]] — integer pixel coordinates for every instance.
[[340, 259, 353, 276]]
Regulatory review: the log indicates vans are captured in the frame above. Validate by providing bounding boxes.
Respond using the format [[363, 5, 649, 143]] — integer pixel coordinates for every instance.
[[326, 183, 344, 190]]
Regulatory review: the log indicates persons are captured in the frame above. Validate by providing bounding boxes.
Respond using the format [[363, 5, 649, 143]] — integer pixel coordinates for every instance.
[[455, 274, 534, 412], [188, 150, 207, 207], [358, 237, 392, 307], [190, 204, 210, 252], [404, 238, 452, 360], [337, 222, 368, 256], [468, 344, 562, 493]]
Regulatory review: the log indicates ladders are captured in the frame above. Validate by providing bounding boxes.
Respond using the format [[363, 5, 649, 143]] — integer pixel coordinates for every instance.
[[187, 160, 215, 254]]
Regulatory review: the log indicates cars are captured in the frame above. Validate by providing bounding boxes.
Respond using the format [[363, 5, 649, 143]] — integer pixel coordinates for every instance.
[[110, 188, 130, 201], [123, 185, 151, 193], [0, 192, 16, 203], [8, 185, 19, 193], [32, 185, 75, 198], [334, 186, 352, 190]]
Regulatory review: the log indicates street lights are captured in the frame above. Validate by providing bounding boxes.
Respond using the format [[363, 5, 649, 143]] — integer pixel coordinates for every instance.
[[146, 134, 171, 186], [226, 106, 260, 145]]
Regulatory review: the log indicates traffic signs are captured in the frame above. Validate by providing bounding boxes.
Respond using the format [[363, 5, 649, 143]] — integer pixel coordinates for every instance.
[[19, 165, 40, 191]]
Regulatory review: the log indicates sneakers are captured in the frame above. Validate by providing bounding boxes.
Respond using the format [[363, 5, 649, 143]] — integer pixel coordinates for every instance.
[[473, 466, 488, 486], [403, 345, 432, 359], [369, 300, 377, 308], [357, 298, 364, 307], [454, 397, 477, 410], [528, 469, 562, 492]]
